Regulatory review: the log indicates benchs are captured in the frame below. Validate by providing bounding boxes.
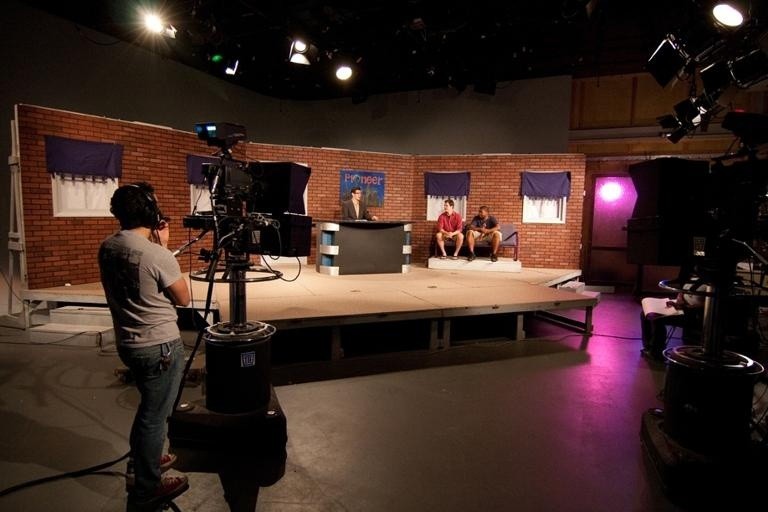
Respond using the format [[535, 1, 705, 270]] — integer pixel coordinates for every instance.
[[435, 223, 518, 261]]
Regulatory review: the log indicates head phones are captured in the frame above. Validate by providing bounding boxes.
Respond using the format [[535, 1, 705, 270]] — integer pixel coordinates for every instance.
[[120, 177, 162, 228]]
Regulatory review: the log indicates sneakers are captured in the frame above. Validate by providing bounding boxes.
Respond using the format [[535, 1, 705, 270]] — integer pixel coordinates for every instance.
[[126, 452, 177, 479], [491, 254, 498, 262], [440, 254, 459, 261], [467, 253, 477, 261], [133, 472, 189, 505]]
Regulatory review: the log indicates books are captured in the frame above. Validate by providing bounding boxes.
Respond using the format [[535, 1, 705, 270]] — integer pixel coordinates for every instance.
[[642, 297, 687, 320]]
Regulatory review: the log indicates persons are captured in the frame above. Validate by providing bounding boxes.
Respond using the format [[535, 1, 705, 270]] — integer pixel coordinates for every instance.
[[639, 250, 709, 360], [341, 186, 378, 222], [435, 199, 464, 259], [96, 180, 195, 506], [466, 206, 503, 262]]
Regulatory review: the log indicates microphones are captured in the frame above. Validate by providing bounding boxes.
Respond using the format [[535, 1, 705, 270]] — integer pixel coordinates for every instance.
[[481, 223, 486, 237]]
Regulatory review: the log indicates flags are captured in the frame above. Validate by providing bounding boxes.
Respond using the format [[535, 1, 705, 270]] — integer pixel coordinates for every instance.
[[339, 168, 386, 208]]
